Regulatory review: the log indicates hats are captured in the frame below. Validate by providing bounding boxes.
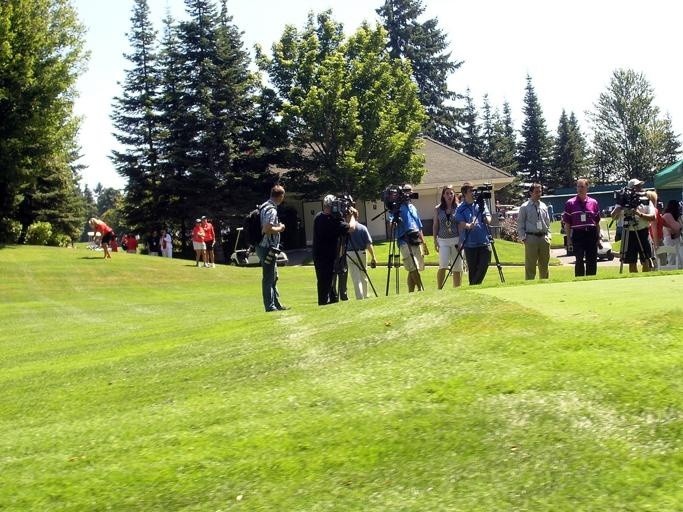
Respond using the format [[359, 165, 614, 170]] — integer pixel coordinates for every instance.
[[628, 178, 645, 188], [195, 215, 207, 224]]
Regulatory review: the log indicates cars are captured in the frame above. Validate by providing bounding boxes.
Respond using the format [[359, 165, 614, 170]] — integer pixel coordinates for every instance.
[[490, 203, 563, 224]]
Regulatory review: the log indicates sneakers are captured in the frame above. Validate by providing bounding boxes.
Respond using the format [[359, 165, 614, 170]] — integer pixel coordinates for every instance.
[[266, 306, 291, 312], [196, 262, 216, 269]]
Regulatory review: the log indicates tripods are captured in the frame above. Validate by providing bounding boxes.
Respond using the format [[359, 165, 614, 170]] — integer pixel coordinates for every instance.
[[440, 199, 505, 290], [325, 216, 378, 302], [372, 202, 424, 296], [608, 205, 651, 274]]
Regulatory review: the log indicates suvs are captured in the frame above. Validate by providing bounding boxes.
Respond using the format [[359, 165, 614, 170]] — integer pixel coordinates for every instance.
[[602, 205, 614, 216]]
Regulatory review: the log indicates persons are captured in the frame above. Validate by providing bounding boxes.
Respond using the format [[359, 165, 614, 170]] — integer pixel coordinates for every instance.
[[85, 214, 140, 259], [517, 182, 553, 281], [456, 192, 464, 204], [157, 228, 171, 257], [452, 182, 492, 286], [191, 218, 208, 268], [430, 186, 463, 290], [146, 230, 159, 257], [311, 192, 357, 305], [386, 184, 427, 293], [328, 236, 349, 304], [546, 201, 554, 222], [560, 178, 682, 276], [254, 183, 292, 313], [198, 215, 216, 268], [343, 207, 377, 300]]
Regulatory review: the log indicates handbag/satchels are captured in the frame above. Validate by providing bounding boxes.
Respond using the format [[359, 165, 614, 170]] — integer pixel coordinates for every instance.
[[407, 231, 423, 247]]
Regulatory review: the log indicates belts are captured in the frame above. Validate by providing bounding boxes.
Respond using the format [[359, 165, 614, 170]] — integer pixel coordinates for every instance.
[[526, 231, 547, 237]]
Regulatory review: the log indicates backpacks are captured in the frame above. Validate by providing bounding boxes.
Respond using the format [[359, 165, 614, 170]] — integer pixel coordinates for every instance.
[[243, 203, 274, 245]]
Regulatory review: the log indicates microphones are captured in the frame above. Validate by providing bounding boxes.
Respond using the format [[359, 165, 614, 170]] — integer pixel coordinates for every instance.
[[455, 244, 464, 260]]
